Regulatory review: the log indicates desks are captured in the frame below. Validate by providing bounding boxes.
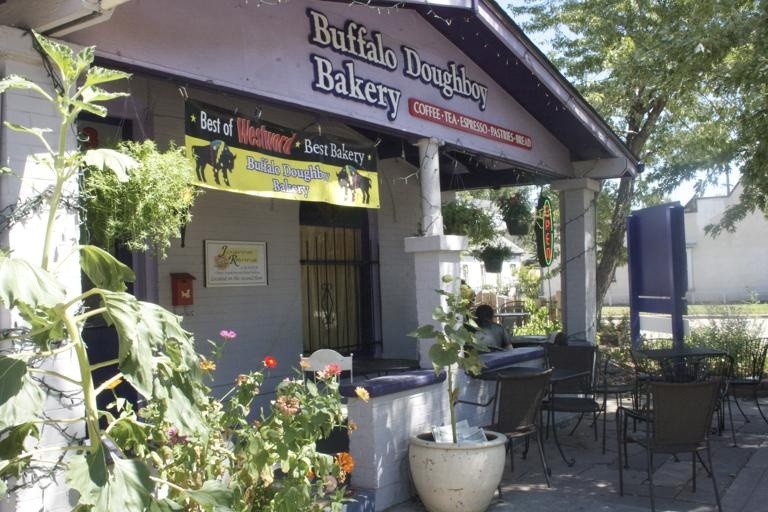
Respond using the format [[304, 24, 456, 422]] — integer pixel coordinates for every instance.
[[353, 357, 418, 380]]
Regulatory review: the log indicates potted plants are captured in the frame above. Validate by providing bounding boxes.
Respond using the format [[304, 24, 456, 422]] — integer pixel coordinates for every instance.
[[479, 193, 535, 273], [409, 275, 511, 511]]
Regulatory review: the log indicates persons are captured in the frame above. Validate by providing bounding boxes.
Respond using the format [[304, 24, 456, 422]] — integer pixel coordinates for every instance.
[[463, 304, 515, 357]]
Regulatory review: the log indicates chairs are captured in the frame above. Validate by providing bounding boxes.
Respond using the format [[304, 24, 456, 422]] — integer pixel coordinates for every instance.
[[453, 343, 768, 512], [297, 349, 354, 389]]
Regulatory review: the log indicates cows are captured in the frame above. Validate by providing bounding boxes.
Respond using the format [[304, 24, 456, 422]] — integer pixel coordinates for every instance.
[[336, 165, 372, 204], [191, 141, 238, 187]]
